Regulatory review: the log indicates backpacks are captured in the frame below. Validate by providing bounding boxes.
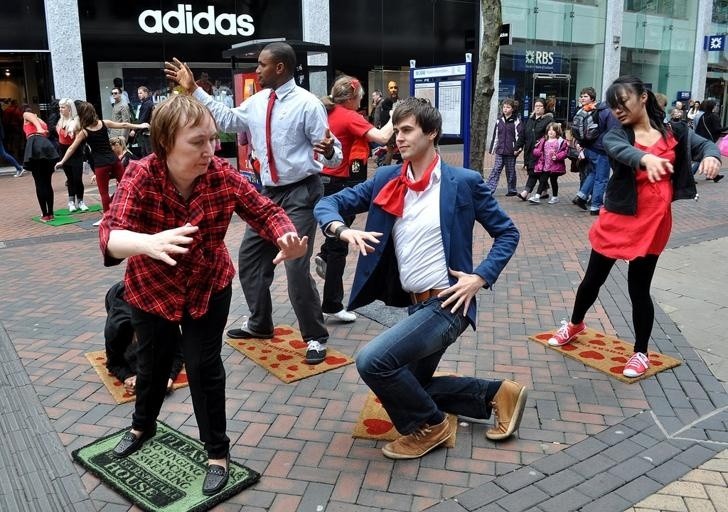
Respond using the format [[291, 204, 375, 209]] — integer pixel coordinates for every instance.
[[570, 101, 608, 141]]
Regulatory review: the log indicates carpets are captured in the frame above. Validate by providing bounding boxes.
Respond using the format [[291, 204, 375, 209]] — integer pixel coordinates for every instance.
[[528, 325, 682, 384], [53, 204, 103, 215], [71, 420, 262, 512], [31, 216, 82, 227], [351, 371, 463, 448], [84, 348, 189, 405], [346, 299, 409, 328], [225, 324, 356, 384]]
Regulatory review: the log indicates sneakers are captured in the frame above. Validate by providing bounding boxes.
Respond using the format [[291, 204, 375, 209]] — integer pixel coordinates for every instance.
[[226, 329, 251, 339], [506, 191, 560, 204], [623, 351, 649, 377], [67, 201, 89, 212], [40, 215, 55, 222], [92, 219, 102, 227], [548, 320, 586, 346], [322, 309, 357, 322], [382, 419, 452, 459], [706, 175, 724, 182], [305, 339, 327, 364], [572, 196, 603, 215], [13, 168, 26, 178], [486, 379, 528, 440], [315, 256, 327, 280]]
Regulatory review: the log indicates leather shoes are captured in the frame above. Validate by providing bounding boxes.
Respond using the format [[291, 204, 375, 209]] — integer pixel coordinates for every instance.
[[112, 423, 157, 458], [202, 453, 230, 495]]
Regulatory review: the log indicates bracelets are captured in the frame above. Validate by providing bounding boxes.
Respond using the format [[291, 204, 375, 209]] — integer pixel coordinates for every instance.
[[333, 225, 350, 238]]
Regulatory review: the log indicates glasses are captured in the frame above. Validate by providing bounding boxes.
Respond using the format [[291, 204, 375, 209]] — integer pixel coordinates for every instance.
[[112, 92, 117, 95]]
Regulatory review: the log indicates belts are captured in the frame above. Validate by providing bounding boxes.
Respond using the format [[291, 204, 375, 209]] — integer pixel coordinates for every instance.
[[409, 288, 447, 305]]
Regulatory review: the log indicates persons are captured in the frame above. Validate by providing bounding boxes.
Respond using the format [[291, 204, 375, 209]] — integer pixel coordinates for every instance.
[[313, 97, 528, 460], [99, 95, 308, 496], [380, 81, 400, 166], [547, 75, 723, 378], [314, 74, 394, 323], [211, 78, 232, 96], [0, 77, 155, 226], [485, 88, 728, 216], [195, 71, 213, 95], [104, 281, 184, 394], [369, 90, 384, 130], [163, 42, 344, 365]]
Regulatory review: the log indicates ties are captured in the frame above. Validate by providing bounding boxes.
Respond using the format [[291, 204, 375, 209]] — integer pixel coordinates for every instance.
[[266, 91, 278, 184]]
